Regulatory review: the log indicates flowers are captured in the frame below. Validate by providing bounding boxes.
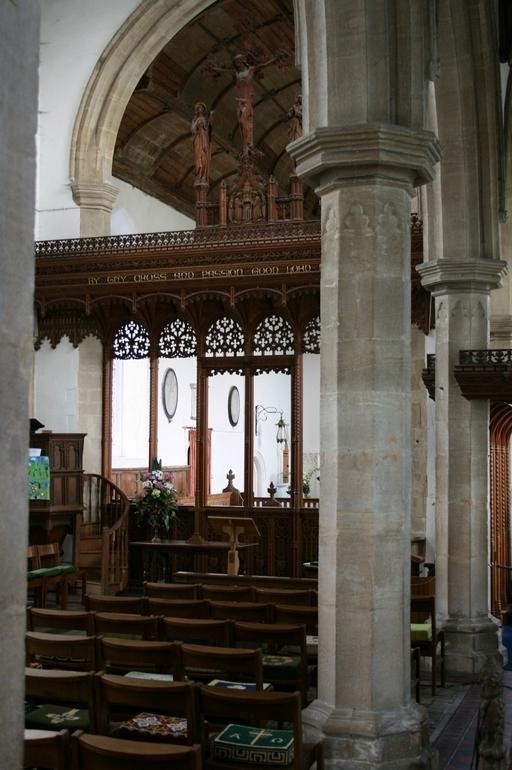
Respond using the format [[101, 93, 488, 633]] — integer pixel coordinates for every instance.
[[133, 455, 179, 533]]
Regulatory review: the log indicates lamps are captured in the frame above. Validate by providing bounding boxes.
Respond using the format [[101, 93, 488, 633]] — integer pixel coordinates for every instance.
[[255, 404, 287, 443]]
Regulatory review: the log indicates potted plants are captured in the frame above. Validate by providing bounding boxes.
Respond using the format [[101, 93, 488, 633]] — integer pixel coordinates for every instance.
[[303, 468, 320, 498]]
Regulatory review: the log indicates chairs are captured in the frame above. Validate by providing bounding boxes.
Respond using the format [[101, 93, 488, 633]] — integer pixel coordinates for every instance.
[[24, 543, 446, 770]]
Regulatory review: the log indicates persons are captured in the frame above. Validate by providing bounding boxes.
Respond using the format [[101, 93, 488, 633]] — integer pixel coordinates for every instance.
[[285, 93, 303, 142], [202, 51, 287, 152], [191, 101, 219, 185]]
